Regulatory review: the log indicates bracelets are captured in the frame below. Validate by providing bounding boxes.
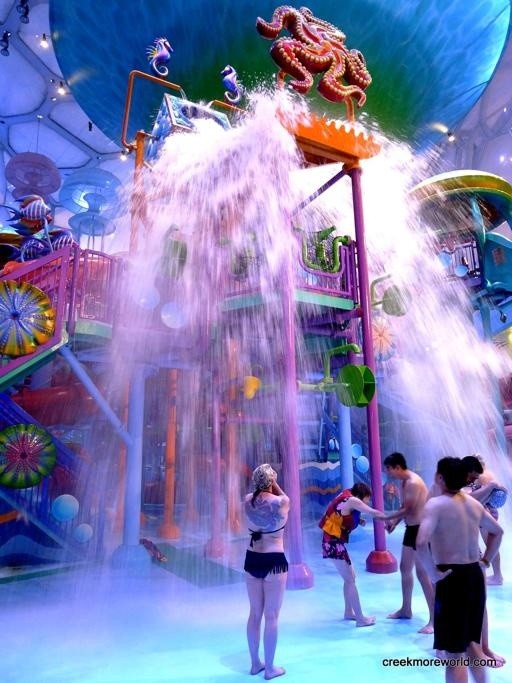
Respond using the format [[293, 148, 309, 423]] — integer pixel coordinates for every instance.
[[481, 557, 491, 567]]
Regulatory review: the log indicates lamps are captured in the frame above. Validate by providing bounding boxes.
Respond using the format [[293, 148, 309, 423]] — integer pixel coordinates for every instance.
[[0, 41, 10, 57], [39, 34, 49, 49], [16, 0, 28, 14], [448, 132, 455, 142], [0, 30, 12, 48], [119, 150, 128, 163], [57, 81, 67, 96], [19, 9, 29, 24]]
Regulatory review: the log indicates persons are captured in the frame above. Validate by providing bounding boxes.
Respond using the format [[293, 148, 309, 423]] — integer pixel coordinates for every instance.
[[383, 452, 438, 633], [462, 452, 504, 584], [415, 454, 503, 682], [318, 481, 409, 626], [429, 455, 508, 668], [243, 463, 291, 679]]
